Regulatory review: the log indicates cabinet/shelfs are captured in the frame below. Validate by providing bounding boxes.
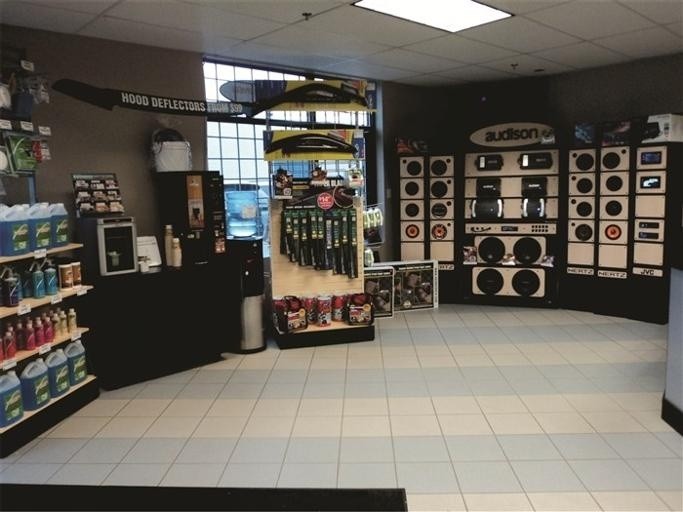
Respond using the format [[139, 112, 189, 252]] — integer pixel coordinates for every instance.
[[0, 241, 99, 461], [69, 260, 227, 394]]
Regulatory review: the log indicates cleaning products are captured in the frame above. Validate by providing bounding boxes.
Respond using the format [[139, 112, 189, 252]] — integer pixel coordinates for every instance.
[[0, 258, 59, 307]]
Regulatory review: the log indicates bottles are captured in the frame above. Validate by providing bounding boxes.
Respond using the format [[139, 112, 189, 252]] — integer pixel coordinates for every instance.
[[0, 307, 78, 360], [163, 224, 174, 266], [170, 237, 182, 268]]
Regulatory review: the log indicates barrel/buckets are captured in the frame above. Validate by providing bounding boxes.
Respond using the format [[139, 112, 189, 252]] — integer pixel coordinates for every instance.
[[224, 184, 262, 238], [1, 200, 71, 256], [45, 347, 71, 398], [64, 339, 88, 386], [20, 358, 52, 411], [150, 141, 194, 173], [0, 369, 24, 427]]
